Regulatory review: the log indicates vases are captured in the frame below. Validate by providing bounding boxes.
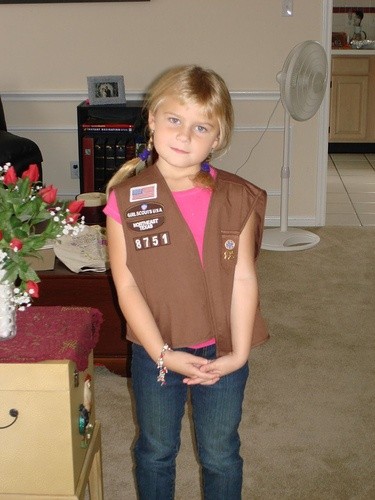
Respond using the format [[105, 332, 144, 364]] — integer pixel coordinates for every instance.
[[0, 282, 18, 341]]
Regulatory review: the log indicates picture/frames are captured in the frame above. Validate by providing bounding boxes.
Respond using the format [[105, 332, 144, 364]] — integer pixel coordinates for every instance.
[[86, 75, 126, 106]]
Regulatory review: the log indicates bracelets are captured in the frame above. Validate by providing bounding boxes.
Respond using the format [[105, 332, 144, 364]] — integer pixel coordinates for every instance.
[[155, 343, 173, 388]]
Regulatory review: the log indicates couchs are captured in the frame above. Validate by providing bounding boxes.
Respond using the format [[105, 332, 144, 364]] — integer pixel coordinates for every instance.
[[0, 95, 43, 186]]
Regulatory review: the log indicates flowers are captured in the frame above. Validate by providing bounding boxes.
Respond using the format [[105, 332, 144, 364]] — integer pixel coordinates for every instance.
[[0, 160, 86, 338]]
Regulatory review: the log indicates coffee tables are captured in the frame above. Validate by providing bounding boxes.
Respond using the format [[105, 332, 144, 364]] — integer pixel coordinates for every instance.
[[20, 256, 131, 377]]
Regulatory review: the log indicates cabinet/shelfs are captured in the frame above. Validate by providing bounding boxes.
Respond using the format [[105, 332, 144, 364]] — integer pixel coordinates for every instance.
[[328, 55, 370, 143], [0, 306, 103, 500]]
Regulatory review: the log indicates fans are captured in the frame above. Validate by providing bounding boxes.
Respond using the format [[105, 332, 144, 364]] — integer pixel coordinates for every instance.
[[256, 39, 328, 251]]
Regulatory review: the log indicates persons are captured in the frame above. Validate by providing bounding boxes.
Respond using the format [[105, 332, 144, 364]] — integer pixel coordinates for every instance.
[[101, 64, 269, 500], [100, 85, 111, 98]]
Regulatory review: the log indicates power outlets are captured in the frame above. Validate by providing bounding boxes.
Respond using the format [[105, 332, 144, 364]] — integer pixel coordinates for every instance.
[[70, 161, 79, 179]]
